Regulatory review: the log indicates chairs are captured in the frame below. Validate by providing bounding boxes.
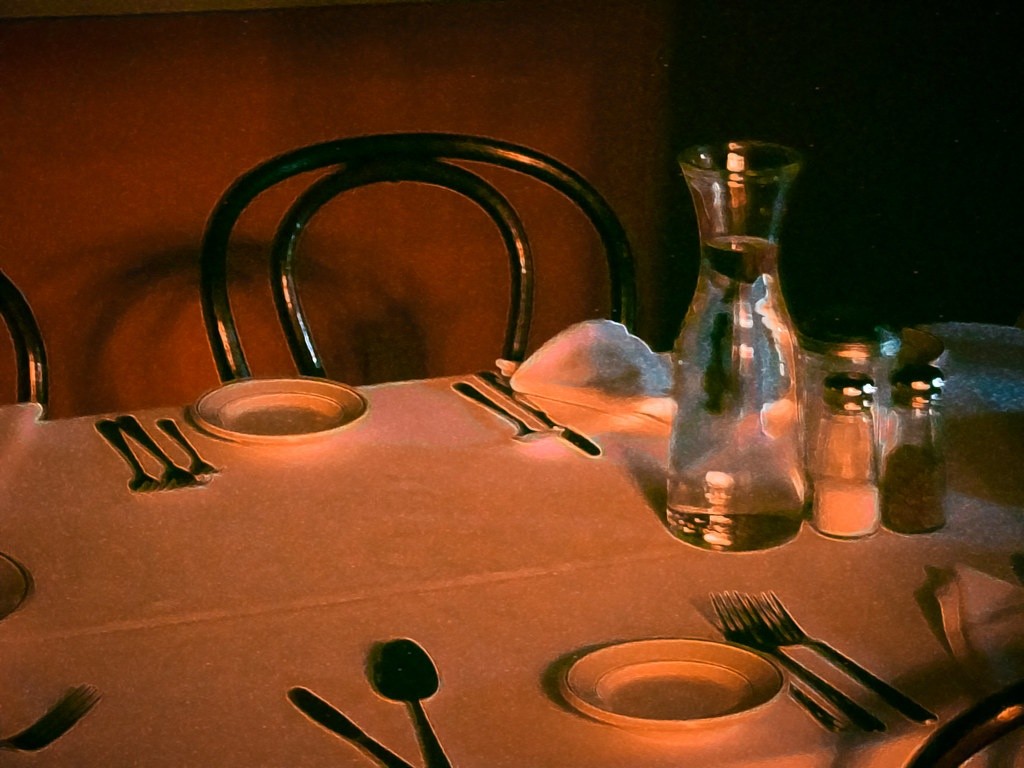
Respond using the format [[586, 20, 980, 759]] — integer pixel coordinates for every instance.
[[192, 132, 633, 385]]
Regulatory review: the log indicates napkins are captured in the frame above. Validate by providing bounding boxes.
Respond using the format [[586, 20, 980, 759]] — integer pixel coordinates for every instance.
[[932, 556, 1024, 702]]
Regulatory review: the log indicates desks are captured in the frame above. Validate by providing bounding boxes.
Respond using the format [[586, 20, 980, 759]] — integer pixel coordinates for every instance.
[[1, 355, 1024, 768]]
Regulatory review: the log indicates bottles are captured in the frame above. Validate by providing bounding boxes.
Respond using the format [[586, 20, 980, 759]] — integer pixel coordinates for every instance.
[[881, 364, 950, 536], [664, 136, 807, 554], [794, 315, 903, 408], [810, 369, 882, 543]]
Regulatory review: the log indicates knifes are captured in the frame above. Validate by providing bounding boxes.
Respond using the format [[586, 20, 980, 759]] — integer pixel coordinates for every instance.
[[286, 686, 414, 768], [473, 370, 602, 459]]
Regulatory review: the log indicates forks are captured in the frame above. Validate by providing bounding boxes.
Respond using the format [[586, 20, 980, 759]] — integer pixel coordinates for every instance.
[[710, 589, 940, 736], [93, 413, 229, 495], [0, 681, 106, 757]]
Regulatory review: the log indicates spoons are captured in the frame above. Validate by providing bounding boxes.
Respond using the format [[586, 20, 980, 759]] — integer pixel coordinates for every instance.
[[451, 380, 566, 444], [368, 636, 455, 768]]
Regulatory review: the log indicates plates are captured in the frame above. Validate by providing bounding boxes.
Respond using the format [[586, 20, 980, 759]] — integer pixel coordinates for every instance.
[[555, 635, 790, 739], [0, 548, 35, 624], [189, 375, 373, 448]]
